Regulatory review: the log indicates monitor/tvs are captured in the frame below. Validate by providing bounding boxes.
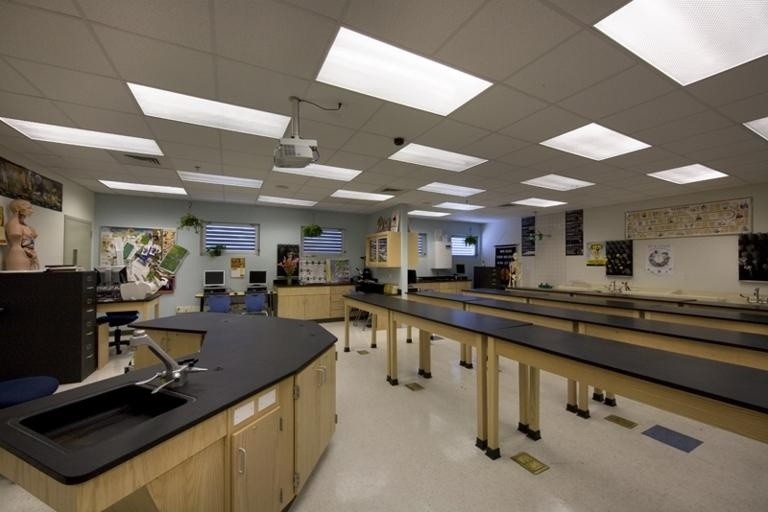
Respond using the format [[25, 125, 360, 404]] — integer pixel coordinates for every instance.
[[204, 270, 225, 286], [248, 270, 267, 286]]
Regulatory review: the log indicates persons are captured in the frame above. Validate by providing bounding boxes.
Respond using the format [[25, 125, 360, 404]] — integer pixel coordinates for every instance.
[[3, 199, 42, 272]]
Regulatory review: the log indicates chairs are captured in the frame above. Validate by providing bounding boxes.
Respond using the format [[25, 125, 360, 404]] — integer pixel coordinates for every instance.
[[206, 292, 268, 318]]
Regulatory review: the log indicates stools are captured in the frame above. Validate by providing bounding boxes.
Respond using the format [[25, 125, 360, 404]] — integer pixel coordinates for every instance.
[[107, 311, 138, 354]]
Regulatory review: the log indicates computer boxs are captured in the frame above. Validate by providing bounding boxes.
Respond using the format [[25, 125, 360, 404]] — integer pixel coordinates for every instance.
[[204, 288, 226, 295], [247, 286, 267, 292]]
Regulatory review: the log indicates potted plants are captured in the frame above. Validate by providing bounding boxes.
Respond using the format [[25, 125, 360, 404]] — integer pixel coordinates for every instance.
[[175, 211, 212, 235], [206, 243, 228, 257]]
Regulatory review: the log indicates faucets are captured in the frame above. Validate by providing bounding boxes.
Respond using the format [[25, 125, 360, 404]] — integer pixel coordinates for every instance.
[[129, 331, 188, 389], [753, 287, 759, 300]]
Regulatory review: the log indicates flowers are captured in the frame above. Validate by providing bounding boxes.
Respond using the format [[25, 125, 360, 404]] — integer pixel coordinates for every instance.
[[277, 250, 300, 277]]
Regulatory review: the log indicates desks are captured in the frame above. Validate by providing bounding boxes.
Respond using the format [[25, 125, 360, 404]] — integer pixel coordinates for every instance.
[[342, 286, 767, 461], [96, 293, 161, 321], [194, 290, 277, 316]]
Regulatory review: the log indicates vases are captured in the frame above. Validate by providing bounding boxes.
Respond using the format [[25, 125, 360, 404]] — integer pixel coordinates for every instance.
[[287, 274, 293, 285]]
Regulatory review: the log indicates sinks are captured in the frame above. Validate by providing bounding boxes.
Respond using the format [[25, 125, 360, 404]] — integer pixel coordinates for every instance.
[[7, 381, 197, 454]]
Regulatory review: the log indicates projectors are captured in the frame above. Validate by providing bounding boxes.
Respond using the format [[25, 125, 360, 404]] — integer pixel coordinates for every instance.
[[273, 139, 317, 168]]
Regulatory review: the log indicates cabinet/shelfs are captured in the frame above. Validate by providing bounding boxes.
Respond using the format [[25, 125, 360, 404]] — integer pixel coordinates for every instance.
[[365, 230, 400, 268], [273, 279, 360, 323], [1, 269, 100, 385], [422, 282, 472, 294]]
